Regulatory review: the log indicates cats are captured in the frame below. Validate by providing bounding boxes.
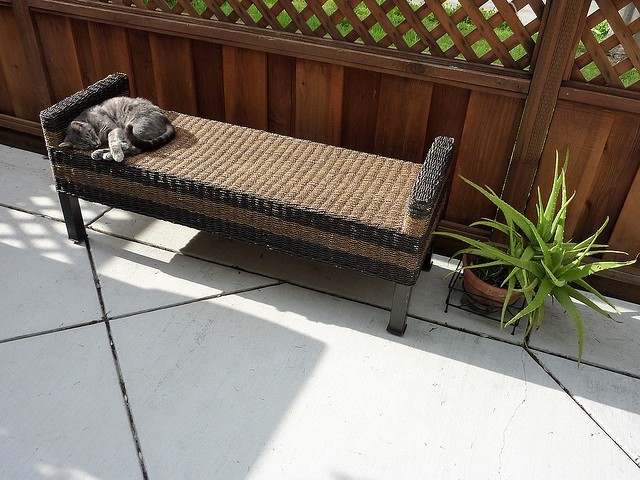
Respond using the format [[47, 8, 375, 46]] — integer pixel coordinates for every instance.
[[58, 95, 176, 164]]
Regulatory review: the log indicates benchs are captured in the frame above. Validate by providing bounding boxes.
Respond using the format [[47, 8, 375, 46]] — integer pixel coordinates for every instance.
[[40, 71, 457, 333]]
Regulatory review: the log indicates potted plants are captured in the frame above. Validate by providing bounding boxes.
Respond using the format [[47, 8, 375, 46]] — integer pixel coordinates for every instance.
[[429, 148, 639, 368]]
